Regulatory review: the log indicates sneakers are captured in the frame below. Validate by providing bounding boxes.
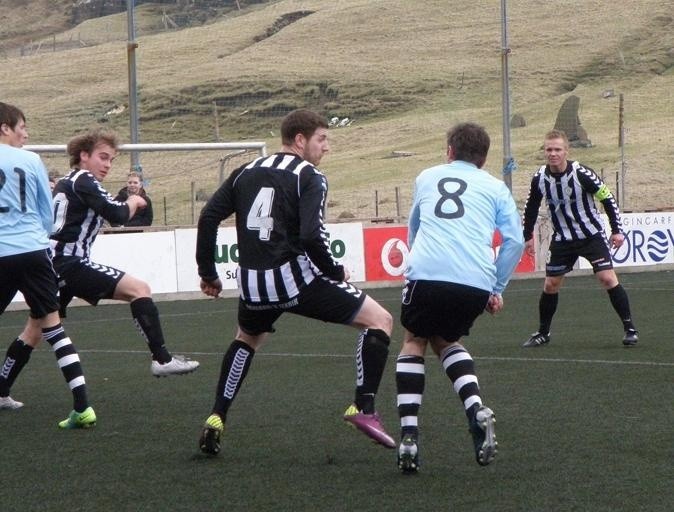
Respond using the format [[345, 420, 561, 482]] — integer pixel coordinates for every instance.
[[623, 329, 639, 345], [524, 332, 550, 347], [399, 435, 419, 473], [58, 407, 96, 429], [199, 415, 223, 455], [343, 404, 396, 448], [151, 356, 199, 378], [469, 407, 498, 466], [1, 397, 24, 409]]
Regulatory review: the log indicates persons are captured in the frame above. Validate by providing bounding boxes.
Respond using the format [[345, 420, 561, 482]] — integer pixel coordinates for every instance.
[[48, 177, 55, 192], [523, 130, 639, 348], [396, 122, 524, 475], [0, 131, 200, 409], [196, 109, 397, 455], [0, 102, 96, 430], [109, 172, 153, 226]]
